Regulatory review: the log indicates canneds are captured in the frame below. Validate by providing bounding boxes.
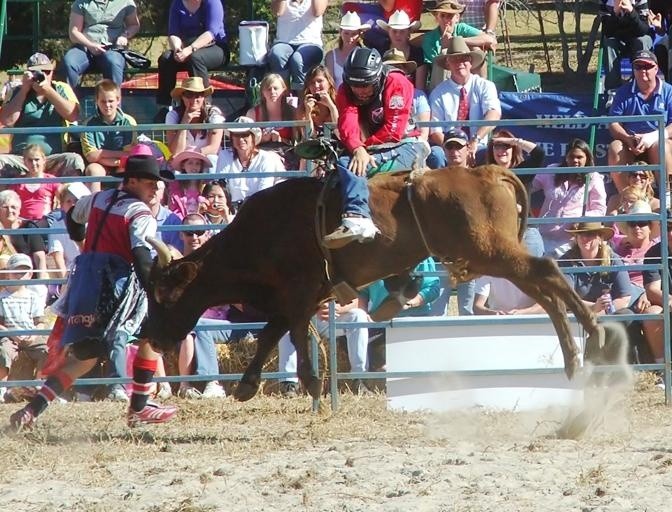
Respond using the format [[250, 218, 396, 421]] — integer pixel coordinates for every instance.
[[603, 293, 615, 315]]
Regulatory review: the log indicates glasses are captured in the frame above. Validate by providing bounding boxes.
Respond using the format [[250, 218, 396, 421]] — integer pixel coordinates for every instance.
[[184, 231, 206, 236]]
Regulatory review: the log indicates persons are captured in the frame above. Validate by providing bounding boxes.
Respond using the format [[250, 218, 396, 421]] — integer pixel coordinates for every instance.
[[556, 222, 635, 316], [0, 0, 549, 403], [529, 135, 608, 254], [599, 0, 672, 371], [8, 153, 182, 433]]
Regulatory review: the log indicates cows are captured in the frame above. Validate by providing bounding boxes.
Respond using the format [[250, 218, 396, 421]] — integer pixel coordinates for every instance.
[[146, 164, 607, 401]]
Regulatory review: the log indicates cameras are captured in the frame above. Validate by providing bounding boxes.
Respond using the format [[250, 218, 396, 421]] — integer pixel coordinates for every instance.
[[308, 92, 321, 102], [206, 202, 219, 211], [31, 71, 46, 83]]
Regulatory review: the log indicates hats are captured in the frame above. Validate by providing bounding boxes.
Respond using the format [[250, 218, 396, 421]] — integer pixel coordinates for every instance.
[[111, 0, 659, 239], [27, 53, 55, 72], [8, 254, 33, 269]]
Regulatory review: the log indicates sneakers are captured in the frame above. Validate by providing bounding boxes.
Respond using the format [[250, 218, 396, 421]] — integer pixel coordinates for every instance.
[[128, 404, 180, 427], [352, 378, 371, 394], [371, 280, 418, 321], [281, 381, 299, 398], [322, 218, 377, 249], [11, 410, 36, 432], [0, 379, 227, 403]]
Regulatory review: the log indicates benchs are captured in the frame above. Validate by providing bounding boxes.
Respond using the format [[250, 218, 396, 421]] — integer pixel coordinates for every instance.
[[596, 41, 661, 165], [3, 58, 265, 124]]
